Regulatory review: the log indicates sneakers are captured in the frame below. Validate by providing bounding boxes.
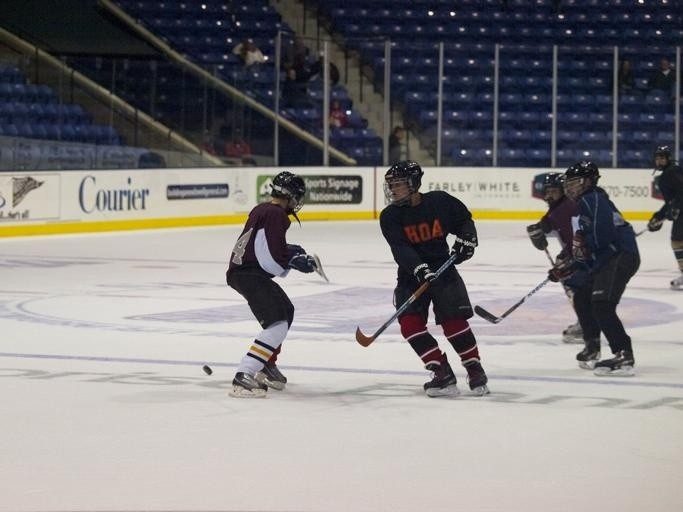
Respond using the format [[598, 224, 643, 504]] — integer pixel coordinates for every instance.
[[260, 359, 287, 383], [232, 372, 268, 392], [562, 318, 587, 337], [670, 273, 683, 286]]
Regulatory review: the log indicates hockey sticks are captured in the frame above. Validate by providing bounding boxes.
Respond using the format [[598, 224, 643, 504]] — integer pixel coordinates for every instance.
[[309, 253, 329, 282], [474, 276, 550, 323], [356, 252, 456, 347], [544, 246, 599, 365]]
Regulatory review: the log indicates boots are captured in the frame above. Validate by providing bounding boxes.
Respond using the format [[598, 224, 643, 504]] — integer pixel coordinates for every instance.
[[594, 343, 635, 367], [461, 357, 488, 390], [422, 352, 457, 391], [576, 335, 602, 360]]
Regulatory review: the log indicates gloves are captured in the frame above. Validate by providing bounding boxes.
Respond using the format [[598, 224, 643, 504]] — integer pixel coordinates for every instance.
[[289, 251, 317, 272], [648, 211, 664, 231], [571, 235, 592, 264], [451, 233, 476, 265], [412, 262, 438, 287], [665, 199, 679, 221], [548, 261, 573, 283], [526, 223, 549, 250]]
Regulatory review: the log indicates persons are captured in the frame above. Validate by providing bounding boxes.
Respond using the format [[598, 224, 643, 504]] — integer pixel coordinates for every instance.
[[379, 160, 487, 391], [563, 161, 640, 368], [612, 60, 635, 93], [650, 146, 683, 287], [330, 101, 356, 153], [218, 126, 255, 167], [224, 171, 318, 389], [233, 38, 341, 108], [532, 173, 604, 337], [388, 126, 406, 164], [648, 59, 676, 91]]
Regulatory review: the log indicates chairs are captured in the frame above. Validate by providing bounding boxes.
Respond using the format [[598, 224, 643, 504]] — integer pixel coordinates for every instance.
[[0, 0, 683, 177]]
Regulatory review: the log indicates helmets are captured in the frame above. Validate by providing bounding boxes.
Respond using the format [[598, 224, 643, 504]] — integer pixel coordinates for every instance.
[[383, 159, 424, 204], [542, 159, 600, 200], [271, 170, 305, 214], [653, 145, 673, 170]]
